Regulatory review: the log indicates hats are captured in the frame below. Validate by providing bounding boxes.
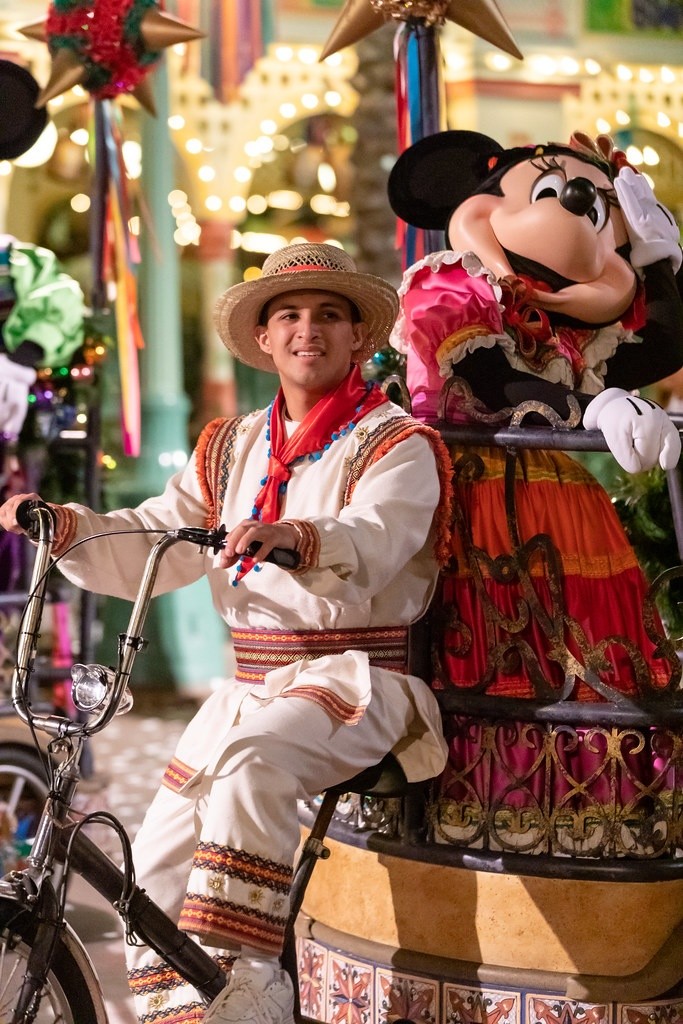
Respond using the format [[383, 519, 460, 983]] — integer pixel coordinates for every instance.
[[212, 242, 400, 375]]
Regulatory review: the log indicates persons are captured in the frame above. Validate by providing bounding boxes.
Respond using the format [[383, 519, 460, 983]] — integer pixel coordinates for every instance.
[[0, 243, 457, 1023]]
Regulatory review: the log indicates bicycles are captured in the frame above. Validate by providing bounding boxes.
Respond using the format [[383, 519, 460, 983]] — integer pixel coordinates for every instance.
[[1, 420, 683, 1024]]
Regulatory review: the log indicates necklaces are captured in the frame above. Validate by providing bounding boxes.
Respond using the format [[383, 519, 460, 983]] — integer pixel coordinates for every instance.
[[231, 380, 378, 586]]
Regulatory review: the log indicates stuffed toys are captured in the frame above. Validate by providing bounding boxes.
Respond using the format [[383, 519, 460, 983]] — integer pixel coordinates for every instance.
[[387, 129, 683, 858]]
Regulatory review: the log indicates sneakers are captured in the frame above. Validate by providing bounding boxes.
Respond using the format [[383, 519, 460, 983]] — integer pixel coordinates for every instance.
[[198, 959, 295, 1024]]
